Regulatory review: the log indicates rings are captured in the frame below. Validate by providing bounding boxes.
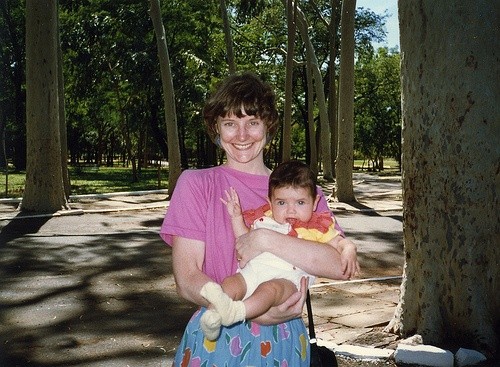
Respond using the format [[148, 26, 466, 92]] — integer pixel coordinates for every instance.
[[236, 256, 242, 261]]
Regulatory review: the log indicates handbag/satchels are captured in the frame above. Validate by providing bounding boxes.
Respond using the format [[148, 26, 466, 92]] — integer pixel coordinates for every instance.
[[306, 289, 338, 367]]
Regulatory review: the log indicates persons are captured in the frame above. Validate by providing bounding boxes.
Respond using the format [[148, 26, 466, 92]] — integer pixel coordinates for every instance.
[[198, 157, 360, 341], [160, 73, 349, 367]]
